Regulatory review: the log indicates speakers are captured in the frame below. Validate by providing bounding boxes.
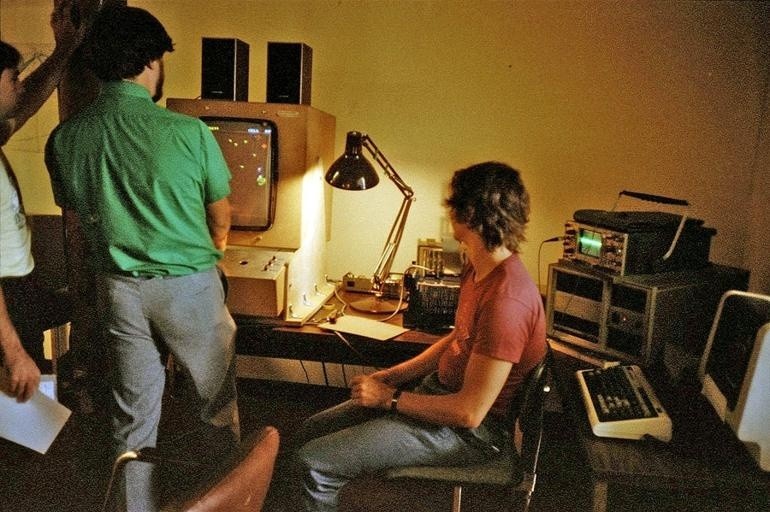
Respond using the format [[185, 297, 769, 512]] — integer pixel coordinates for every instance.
[[201, 36, 249, 102], [266, 41, 313, 105]]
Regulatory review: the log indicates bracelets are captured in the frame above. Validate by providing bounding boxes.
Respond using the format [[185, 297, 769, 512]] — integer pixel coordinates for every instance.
[[391, 391, 401, 413]]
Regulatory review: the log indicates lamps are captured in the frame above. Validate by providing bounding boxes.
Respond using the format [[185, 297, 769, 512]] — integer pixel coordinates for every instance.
[[325, 131, 414, 315]]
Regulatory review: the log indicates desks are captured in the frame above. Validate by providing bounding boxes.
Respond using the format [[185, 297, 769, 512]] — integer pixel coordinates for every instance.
[[167, 281, 452, 370], [547, 353, 770, 512]]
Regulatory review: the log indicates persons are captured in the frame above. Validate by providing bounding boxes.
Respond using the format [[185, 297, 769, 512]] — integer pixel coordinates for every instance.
[[292, 162, 546, 512], [0, 1, 88, 402], [43, 6, 242, 512]]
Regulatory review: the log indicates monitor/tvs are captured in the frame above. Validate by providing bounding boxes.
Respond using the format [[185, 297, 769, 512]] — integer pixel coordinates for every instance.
[[164, 97, 336, 328]]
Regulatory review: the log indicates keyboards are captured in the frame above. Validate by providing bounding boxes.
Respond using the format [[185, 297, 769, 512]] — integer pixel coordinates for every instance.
[[576, 366, 672, 443]]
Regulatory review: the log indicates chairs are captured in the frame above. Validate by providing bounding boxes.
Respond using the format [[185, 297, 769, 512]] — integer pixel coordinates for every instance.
[[101, 426, 280, 511], [385, 362, 552, 510]]
